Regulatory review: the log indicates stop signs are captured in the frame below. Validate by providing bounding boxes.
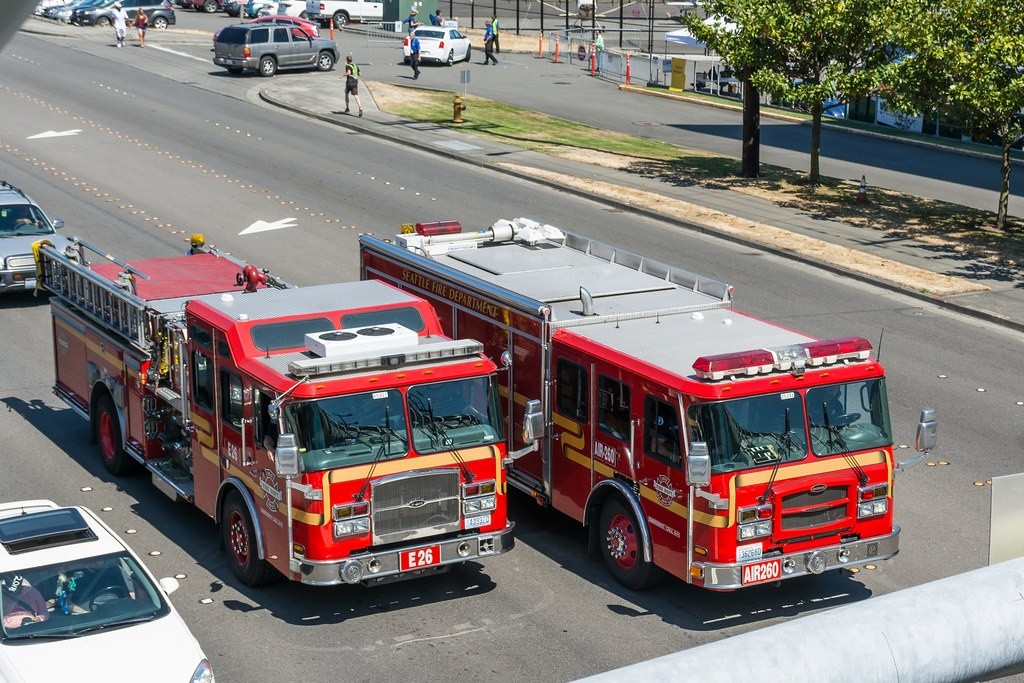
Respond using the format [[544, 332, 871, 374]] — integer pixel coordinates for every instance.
[[577, 45, 586, 61]]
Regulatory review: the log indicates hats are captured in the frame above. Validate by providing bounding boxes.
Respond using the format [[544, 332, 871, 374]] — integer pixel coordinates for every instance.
[[116, 4, 122, 8]]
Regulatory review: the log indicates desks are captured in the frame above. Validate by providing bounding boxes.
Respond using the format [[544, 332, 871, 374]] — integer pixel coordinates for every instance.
[[698, 77, 740, 91]]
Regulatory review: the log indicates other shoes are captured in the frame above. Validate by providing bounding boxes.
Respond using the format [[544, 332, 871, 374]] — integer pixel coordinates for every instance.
[[359, 107, 363, 118], [483, 60, 488, 65], [117, 43, 124, 47], [141, 43, 145, 47], [345, 108, 351, 115], [414, 71, 421, 80], [494, 60, 498, 65]]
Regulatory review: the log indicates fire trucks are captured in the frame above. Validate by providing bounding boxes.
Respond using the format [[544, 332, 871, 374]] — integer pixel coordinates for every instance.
[[30, 234, 546, 589], [359, 216, 941, 595]]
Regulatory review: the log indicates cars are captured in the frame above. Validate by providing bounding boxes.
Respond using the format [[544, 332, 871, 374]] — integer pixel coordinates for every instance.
[[0, 499, 215, 681], [244, 0, 269, 18], [174, 0, 191, 9], [186, 0, 223, 13], [260, 0, 278, 18], [402, 26, 472, 66], [33, 0, 112, 28], [213, 15, 320, 42], [80, 0, 177, 31], [276, 0, 312, 23], [222, 0, 250, 18]]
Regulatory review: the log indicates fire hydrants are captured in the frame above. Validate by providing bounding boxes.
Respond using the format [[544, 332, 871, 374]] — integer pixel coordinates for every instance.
[[449, 94, 468, 123]]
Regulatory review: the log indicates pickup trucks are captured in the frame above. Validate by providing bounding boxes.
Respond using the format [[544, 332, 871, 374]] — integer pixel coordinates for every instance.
[[305, 0, 383, 27]]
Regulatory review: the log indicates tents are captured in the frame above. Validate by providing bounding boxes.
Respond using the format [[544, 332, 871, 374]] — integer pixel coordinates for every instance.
[[664, 13, 745, 100]]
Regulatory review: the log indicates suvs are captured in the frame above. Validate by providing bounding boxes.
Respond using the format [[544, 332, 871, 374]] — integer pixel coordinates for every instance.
[[212, 23, 341, 76], [0, 181, 76, 293]]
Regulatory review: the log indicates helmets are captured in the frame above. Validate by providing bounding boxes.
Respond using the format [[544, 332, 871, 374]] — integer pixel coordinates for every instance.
[[190, 234, 204, 247]]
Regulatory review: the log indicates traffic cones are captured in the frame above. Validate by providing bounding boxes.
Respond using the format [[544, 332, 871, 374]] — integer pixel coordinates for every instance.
[[853, 175, 872, 203]]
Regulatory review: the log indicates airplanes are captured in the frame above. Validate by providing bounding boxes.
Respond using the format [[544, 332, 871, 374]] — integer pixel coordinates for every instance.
[[538, 0, 637, 31]]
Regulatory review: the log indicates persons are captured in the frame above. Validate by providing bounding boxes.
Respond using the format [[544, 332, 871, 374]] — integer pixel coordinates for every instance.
[[63, 560, 137, 615], [808, 386, 844, 429], [9, 207, 43, 227], [490, 14, 500, 54], [650, 408, 723, 463], [408, 9, 420, 36], [409, 31, 421, 79], [483, 20, 498, 65], [110, 2, 131, 48], [433, 10, 443, 26], [339, 56, 363, 117], [263, 421, 276, 462], [594, 30, 604, 72], [132, 8, 149, 48], [2, 573, 51, 629]]
